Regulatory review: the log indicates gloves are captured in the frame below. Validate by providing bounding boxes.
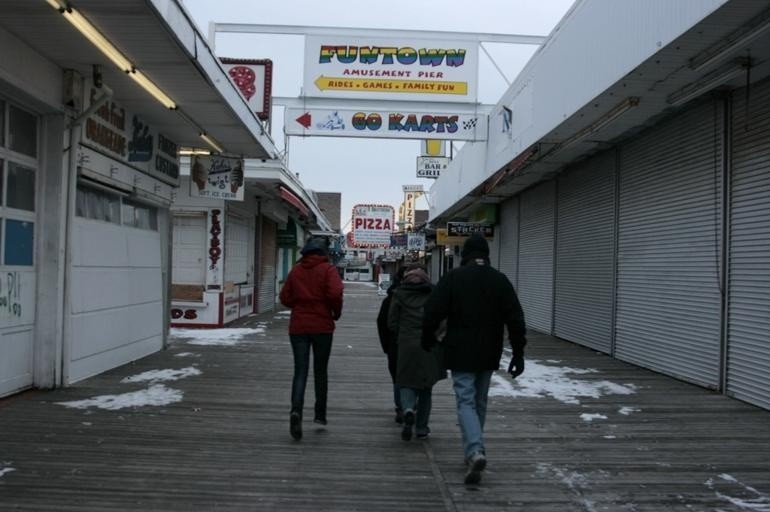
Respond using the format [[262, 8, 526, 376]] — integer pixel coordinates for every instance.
[[508, 355, 525, 378], [421, 330, 438, 352]]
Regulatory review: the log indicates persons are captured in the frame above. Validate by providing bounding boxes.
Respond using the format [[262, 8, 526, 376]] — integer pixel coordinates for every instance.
[[387, 262, 448, 441], [421, 230, 528, 484], [279, 234, 344, 440], [376, 265, 404, 425]]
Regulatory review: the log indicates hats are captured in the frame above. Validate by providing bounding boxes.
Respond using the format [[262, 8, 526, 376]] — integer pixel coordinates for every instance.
[[461, 234, 490, 266]]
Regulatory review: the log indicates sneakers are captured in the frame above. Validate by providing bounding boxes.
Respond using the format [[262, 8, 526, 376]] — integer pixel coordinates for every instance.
[[395, 407, 431, 440], [463, 450, 486, 484], [314, 416, 328, 425], [290, 409, 302, 441]]
